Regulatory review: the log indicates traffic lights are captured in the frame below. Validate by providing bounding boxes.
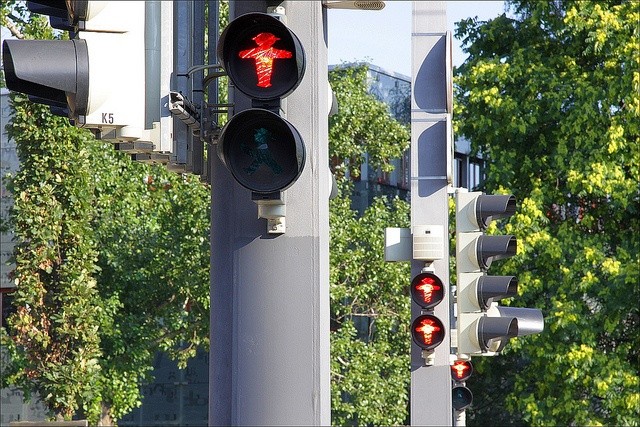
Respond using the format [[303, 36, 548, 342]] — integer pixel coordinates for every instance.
[[452, 358, 474, 410], [409, 270, 445, 349], [487, 303, 545, 349], [4, 1, 91, 128], [216, 9, 306, 198], [454, 189, 519, 353]]
